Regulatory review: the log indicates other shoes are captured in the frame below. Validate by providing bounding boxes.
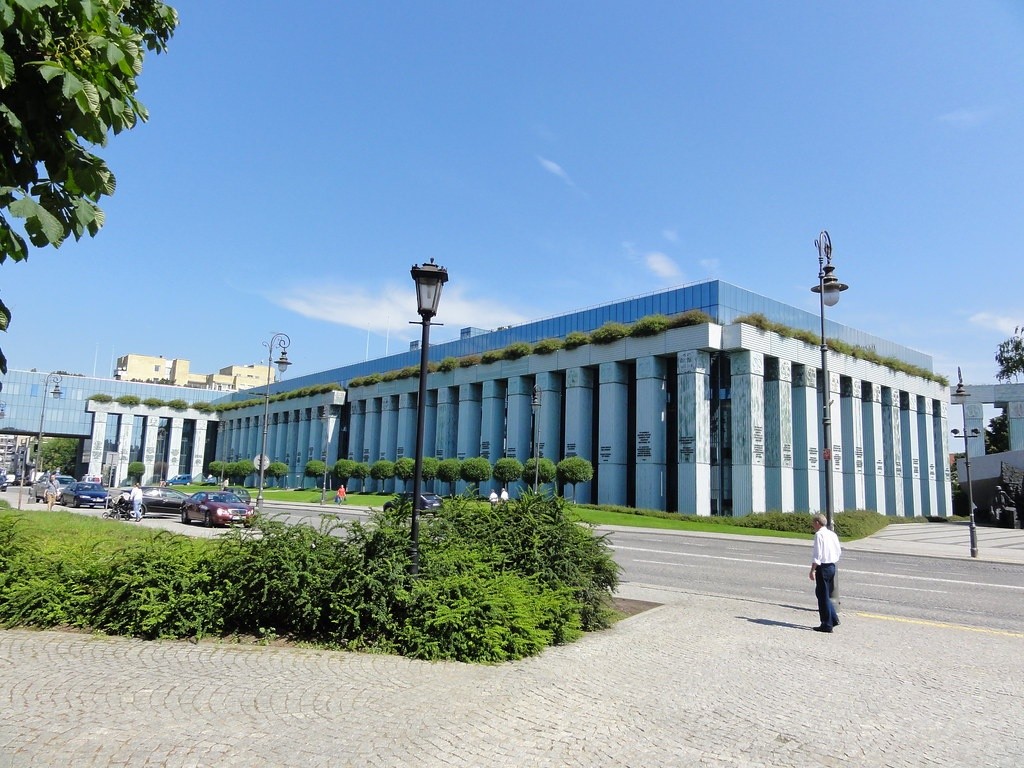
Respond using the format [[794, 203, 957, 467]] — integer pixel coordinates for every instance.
[[833, 619, 840, 626], [813, 627, 833, 632]]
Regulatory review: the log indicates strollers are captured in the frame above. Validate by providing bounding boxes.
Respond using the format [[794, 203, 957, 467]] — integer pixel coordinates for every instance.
[[103, 493, 130, 521]]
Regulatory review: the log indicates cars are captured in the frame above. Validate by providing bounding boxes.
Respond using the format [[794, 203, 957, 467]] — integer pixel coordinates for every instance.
[[60, 482, 107, 508], [224, 486, 251, 504], [180, 491, 254, 528], [383, 492, 445, 517], [0, 475, 7, 492], [124, 487, 189, 516], [32, 475, 77, 504]]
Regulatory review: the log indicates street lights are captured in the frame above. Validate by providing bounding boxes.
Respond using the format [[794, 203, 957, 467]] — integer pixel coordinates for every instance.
[[257, 333, 292, 515], [810, 232, 849, 613], [29, 373, 62, 501], [406, 258, 448, 586], [320, 406, 337, 504], [532, 385, 541, 497], [951, 367, 980, 558]]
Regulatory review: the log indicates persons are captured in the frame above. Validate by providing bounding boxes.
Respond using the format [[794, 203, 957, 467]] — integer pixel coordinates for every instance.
[[990, 485, 1016, 528], [81, 473, 97, 482], [809, 514, 842, 634], [159, 480, 167, 487], [500, 488, 509, 502], [336, 484, 346, 507], [46, 474, 59, 511], [207, 474, 213, 479], [489, 489, 499, 507], [223, 477, 229, 491], [130, 483, 143, 522]]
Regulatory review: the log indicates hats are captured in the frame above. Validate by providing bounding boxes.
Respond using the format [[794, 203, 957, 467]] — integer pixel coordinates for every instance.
[[502, 488, 505, 491]]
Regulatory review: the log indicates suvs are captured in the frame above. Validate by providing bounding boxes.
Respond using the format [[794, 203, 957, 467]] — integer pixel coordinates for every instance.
[[166, 475, 192, 486]]
